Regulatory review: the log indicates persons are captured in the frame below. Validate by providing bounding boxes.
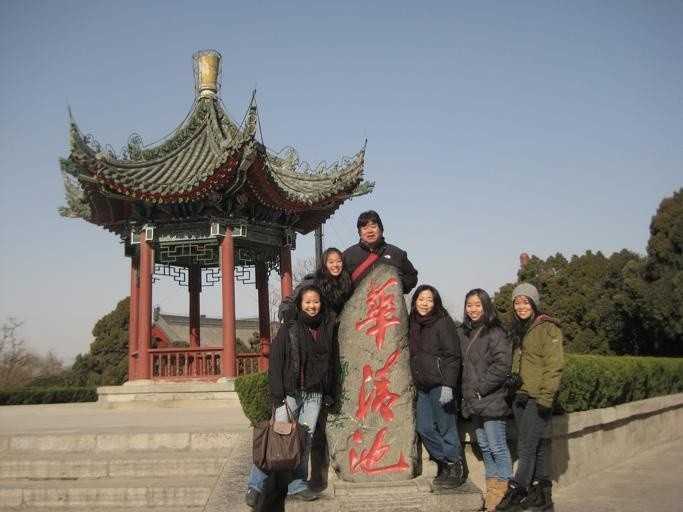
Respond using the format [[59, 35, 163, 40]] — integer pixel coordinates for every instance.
[[279, 247, 353, 327], [408, 284, 469, 490], [455, 288, 515, 511], [344, 210, 418, 295], [246, 285, 333, 509], [489, 282, 566, 512]]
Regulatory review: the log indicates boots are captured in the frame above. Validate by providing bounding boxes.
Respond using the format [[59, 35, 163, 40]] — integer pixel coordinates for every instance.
[[483, 479, 497, 512], [494, 480, 527, 512], [494, 480, 507, 512], [510, 481, 553, 512]]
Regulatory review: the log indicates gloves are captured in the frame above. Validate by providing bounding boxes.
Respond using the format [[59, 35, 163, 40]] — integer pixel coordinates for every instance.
[[322, 396, 335, 407], [536, 404, 552, 420], [271, 396, 287, 408], [438, 386, 454, 406]]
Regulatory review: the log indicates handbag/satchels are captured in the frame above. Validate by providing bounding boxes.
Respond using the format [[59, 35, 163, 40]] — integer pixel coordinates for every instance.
[[250, 398, 309, 472]]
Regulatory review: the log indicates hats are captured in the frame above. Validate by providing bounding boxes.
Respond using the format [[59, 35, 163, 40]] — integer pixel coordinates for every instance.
[[511, 283, 540, 313]]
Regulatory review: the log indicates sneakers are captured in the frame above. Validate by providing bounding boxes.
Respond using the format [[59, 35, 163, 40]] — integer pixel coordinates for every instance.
[[440, 461, 465, 488], [432, 463, 449, 484], [244, 486, 258, 507], [287, 487, 317, 500]]
